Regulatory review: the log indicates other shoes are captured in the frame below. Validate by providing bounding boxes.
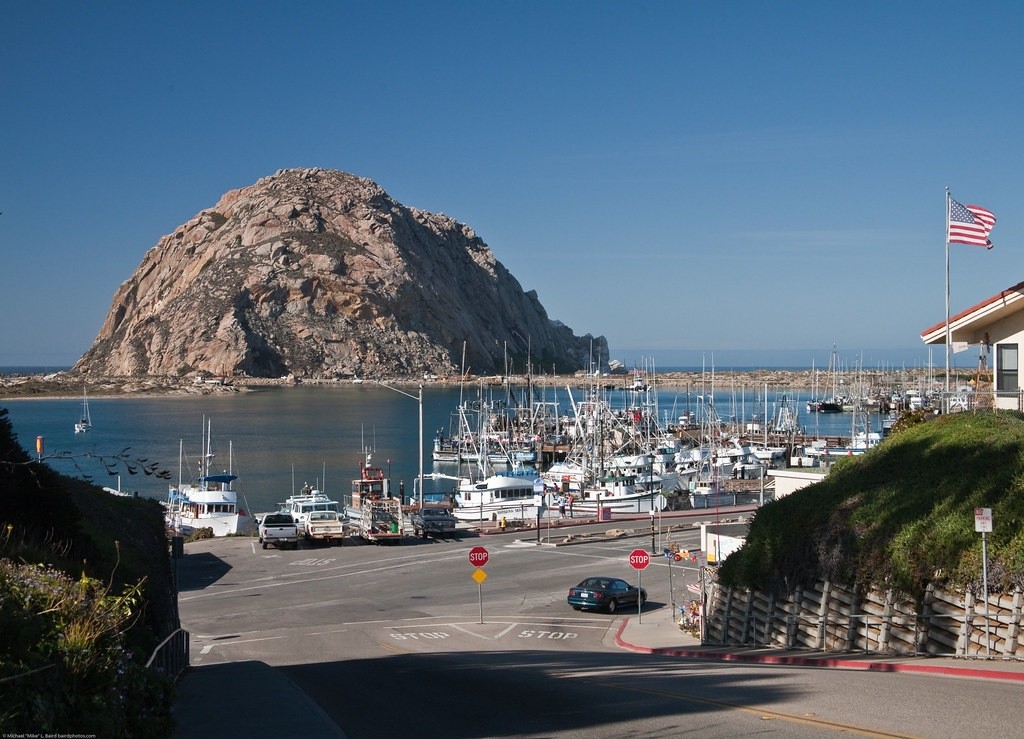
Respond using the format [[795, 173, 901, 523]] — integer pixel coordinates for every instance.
[[558, 517, 567, 519], [569, 517, 572, 520]]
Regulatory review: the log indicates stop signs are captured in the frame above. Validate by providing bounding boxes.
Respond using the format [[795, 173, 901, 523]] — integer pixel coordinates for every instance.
[[468, 546, 489, 568], [628, 549, 650, 571]]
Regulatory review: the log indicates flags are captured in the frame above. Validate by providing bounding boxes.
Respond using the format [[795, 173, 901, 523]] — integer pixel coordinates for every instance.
[[948, 196, 996, 249]]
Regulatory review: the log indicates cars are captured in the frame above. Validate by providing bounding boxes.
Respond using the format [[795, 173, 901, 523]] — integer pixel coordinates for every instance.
[[566, 576, 647, 613]]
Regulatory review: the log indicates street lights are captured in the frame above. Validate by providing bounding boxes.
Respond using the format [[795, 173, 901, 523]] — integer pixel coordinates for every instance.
[[646, 451, 657, 556], [354, 378, 424, 511]]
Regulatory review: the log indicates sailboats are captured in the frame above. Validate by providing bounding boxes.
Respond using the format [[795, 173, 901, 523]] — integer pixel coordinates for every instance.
[[409, 336, 941, 526], [73, 386, 93, 433]]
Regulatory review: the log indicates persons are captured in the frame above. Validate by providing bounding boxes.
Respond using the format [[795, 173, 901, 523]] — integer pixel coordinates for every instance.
[[239, 508, 245, 515], [304, 482, 308, 487], [566, 494, 574, 519], [558, 494, 566, 519], [552, 483, 558, 492], [501, 517, 506, 531]]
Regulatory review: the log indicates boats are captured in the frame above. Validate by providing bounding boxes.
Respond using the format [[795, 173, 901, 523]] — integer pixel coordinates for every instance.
[[285, 460, 345, 534], [345, 423, 405, 517], [159, 414, 239, 537], [103, 475, 131, 497]]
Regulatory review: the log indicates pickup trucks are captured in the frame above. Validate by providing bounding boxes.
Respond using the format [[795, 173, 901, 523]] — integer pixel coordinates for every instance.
[[255, 513, 299, 551], [304, 510, 343, 544], [411, 507, 456, 541]]
